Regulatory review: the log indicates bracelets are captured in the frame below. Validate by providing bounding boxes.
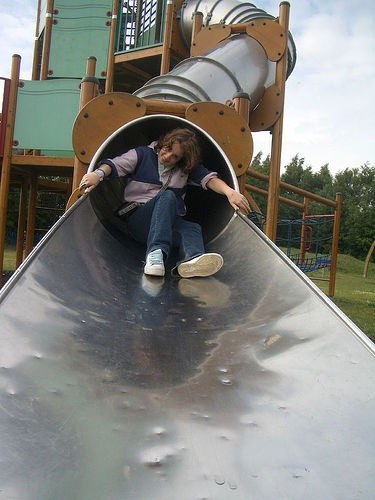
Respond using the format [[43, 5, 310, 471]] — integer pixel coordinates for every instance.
[[94, 167, 106, 182]]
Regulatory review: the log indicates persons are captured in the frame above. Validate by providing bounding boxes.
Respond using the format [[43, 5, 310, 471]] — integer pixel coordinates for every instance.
[[79, 128, 252, 278]]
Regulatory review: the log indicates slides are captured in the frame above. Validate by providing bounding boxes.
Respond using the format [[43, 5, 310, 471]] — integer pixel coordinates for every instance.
[[0, 0, 375, 500]]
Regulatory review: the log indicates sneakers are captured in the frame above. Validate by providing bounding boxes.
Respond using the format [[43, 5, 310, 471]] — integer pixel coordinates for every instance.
[[144, 249, 166, 277], [177, 253, 224, 278]]
